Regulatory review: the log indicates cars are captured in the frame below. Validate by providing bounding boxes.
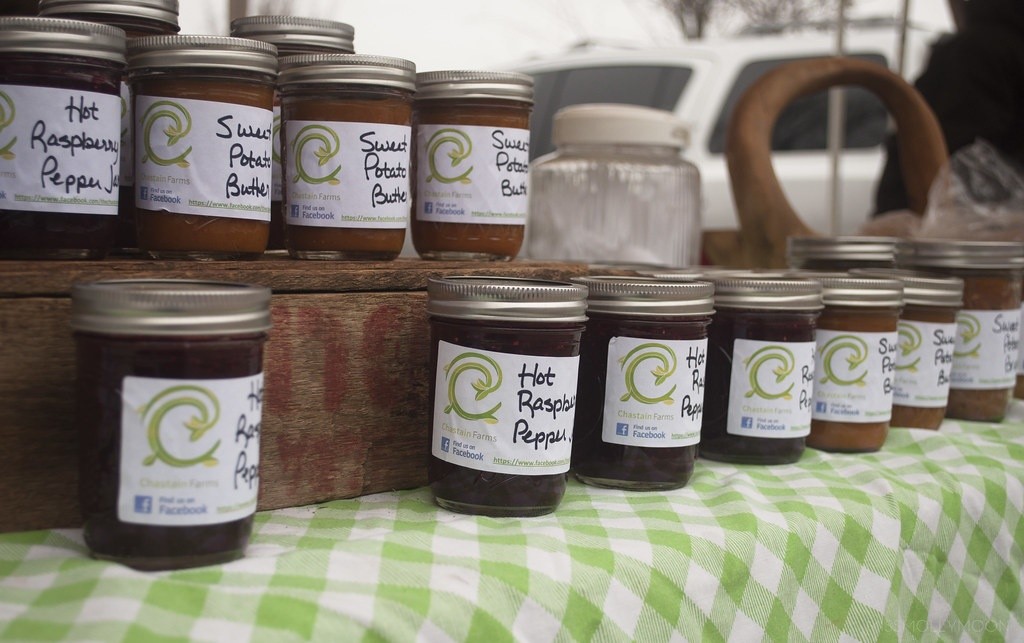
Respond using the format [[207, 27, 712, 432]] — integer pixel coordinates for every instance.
[[517, 40, 897, 248]]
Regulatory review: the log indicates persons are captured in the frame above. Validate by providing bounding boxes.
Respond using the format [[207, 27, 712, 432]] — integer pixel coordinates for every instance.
[[858, 0, 1024, 239]]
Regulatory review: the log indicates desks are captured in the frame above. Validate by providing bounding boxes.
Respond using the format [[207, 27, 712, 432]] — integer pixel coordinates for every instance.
[[0, 261, 1024, 643]]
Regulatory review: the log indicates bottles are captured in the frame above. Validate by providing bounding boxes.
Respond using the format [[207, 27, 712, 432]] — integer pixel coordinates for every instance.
[[526, 102, 701, 270]]
